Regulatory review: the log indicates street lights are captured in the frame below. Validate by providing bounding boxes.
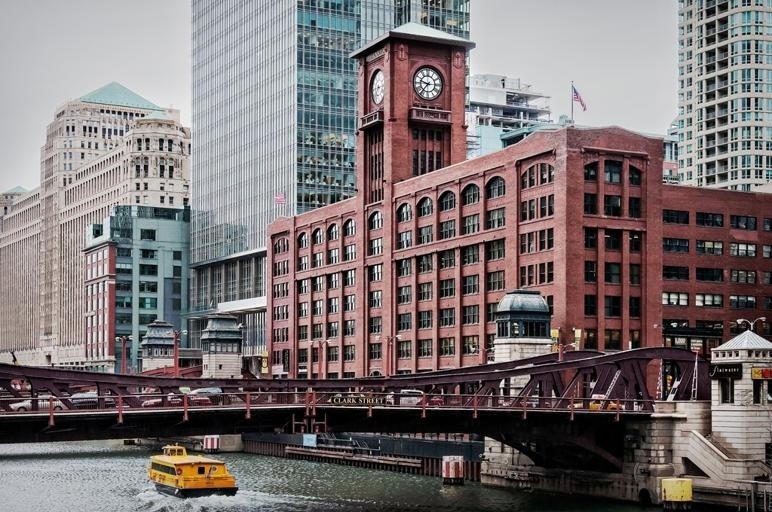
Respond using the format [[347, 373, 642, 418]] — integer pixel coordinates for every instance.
[[545, 343, 577, 361], [165, 329, 188, 376], [115, 335, 134, 374], [736, 317, 766, 332], [376, 335, 403, 374], [308, 339, 332, 379], [469, 347, 495, 364]]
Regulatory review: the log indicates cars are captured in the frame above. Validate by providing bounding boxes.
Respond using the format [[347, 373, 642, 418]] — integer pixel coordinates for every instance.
[[427, 396, 444, 406], [142, 394, 213, 408], [9, 394, 73, 411], [567, 393, 622, 411], [503, 394, 551, 408]]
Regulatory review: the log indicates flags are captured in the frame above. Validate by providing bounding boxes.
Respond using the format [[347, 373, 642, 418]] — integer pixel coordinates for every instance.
[[572, 85, 586, 112]]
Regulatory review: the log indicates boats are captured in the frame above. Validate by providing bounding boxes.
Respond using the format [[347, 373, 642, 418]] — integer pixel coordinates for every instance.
[[149, 442, 239, 498]]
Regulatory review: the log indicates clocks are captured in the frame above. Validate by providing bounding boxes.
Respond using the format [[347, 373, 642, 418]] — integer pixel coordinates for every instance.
[[413, 68, 443, 100], [372, 70, 385, 104]]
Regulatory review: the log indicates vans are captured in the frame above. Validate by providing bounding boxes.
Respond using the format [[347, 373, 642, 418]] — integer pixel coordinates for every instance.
[[189, 387, 223, 404], [384, 389, 426, 404], [69, 392, 116, 409]]
